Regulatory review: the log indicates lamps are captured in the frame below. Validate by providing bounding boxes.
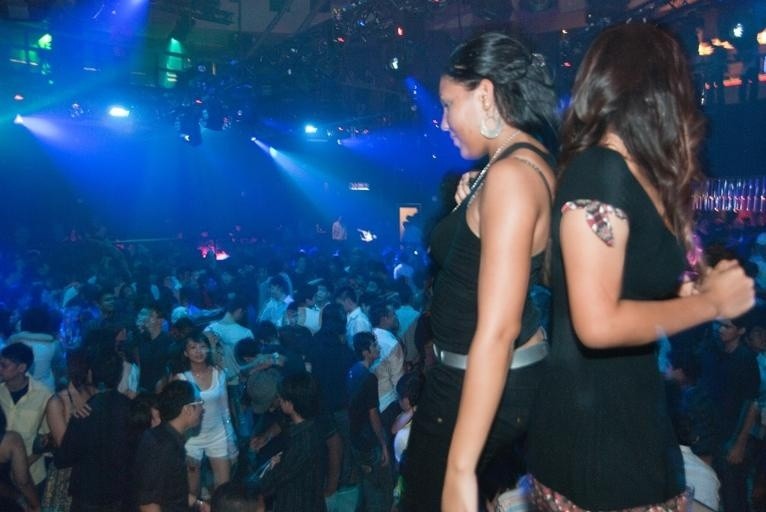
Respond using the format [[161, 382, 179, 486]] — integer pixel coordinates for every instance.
[[175, 95, 227, 151]]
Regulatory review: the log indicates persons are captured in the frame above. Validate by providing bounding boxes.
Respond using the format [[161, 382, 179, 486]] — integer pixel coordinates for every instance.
[[526, 21, 757, 512], [663, 207, 766, 512], [1, 216, 435, 512], [398, 31, 566, 512]]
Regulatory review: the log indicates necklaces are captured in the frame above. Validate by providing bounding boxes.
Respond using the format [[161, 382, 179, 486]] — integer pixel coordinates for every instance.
[[449, 128, 522, 213]]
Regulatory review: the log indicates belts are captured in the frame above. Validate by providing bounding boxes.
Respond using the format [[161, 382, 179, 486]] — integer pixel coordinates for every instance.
[[431, 340, 552, 372]]
